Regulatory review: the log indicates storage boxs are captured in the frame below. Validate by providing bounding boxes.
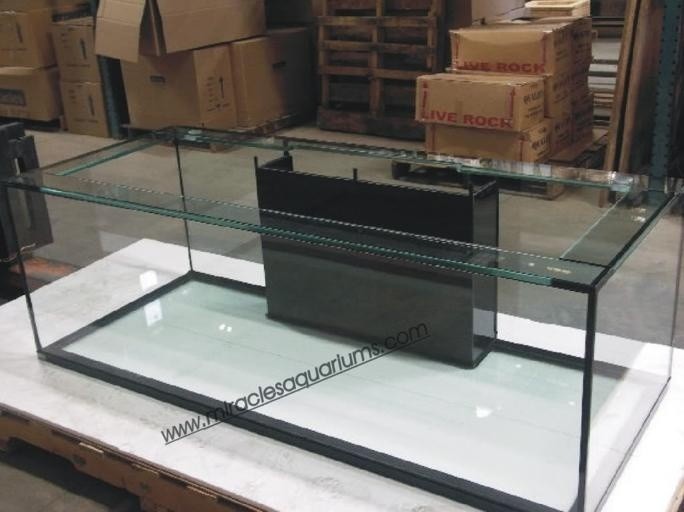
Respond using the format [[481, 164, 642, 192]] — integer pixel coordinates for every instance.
[[2, 1, 317, 133], [413, 0, 604, 169]]
